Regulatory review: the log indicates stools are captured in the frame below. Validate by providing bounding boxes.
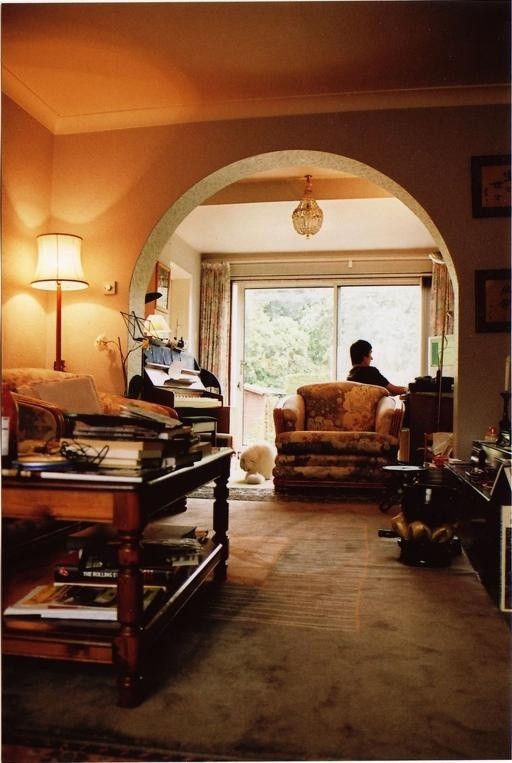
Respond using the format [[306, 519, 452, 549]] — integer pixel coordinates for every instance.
[[196, 432, 233, 448]]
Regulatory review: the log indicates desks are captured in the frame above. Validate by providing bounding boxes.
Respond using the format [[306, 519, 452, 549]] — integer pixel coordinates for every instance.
[[400, 388, 454, 466]]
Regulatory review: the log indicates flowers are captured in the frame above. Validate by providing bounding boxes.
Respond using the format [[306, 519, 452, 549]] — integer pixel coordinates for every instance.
[[94, 332, 153, 396]]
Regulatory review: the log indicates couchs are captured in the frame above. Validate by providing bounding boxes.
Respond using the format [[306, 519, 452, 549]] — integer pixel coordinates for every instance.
[[0, 369, 179, 439], [272, 382, 406, 501]]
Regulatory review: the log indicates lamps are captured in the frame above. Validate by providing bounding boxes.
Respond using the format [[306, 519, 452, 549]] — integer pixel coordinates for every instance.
[[28, 232, 90, 371], [292, 174, 323, 241]]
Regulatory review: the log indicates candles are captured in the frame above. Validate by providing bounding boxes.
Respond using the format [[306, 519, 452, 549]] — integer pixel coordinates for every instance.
[[504, 353, 510, 393]]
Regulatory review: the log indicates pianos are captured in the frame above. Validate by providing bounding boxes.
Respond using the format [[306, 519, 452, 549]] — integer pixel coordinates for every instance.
[[140, 360, 224, 436]]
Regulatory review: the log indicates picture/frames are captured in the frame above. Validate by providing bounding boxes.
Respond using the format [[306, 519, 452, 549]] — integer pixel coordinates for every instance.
[[475, 268, 512, 332], [469, 154, 512, 220], [155, 259, 170, 312]]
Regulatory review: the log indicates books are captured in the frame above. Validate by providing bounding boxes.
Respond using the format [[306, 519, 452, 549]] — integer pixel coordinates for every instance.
[[5, 525, 209, 621], [60, 406, 210, 471]]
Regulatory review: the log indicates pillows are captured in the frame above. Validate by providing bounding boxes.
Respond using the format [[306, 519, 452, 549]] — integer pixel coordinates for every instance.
[[14, 374, 105, 416]]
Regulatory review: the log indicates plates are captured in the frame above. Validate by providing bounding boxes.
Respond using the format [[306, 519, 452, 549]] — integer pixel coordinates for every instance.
[[17, 456, 70, 467]]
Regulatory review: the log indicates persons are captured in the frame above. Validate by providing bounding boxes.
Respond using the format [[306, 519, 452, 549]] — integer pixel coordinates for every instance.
[[346, 340, 409, 394]]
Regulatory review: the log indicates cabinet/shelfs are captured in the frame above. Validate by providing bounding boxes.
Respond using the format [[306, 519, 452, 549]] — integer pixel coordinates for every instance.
[[447, 440, 512, 614]]
